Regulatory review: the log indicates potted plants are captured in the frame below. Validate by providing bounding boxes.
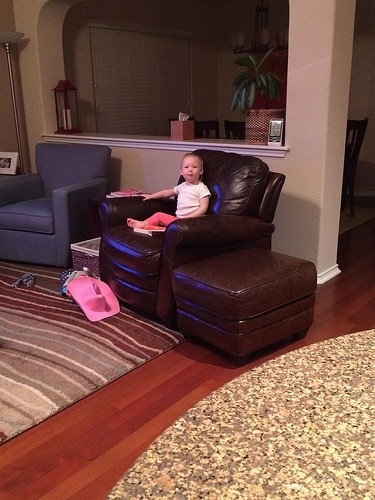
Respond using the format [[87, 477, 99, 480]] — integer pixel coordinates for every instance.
[[229, 47, 285, 146]]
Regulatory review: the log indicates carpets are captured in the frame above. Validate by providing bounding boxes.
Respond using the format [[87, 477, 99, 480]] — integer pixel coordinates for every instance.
[[0, 259, 187, 445], [339, 206, 375, 236]]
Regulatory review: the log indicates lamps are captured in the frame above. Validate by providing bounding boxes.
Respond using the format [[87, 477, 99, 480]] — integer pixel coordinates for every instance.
[[0, 31, 27, 175], [50, 79, 84, 134]]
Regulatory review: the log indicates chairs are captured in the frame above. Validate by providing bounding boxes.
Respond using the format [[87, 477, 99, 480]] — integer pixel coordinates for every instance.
[[339, 117, 369, 223], [197, 119, 220, 139], [224, 120, 246, 140]]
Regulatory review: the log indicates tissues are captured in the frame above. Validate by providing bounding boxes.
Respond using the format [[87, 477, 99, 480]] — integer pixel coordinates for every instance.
[[170, 112, 194, 140]]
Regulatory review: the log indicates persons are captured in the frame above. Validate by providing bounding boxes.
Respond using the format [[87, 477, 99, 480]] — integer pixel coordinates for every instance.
[[127, 153, 212, 230], [1, 158, 9, 168]]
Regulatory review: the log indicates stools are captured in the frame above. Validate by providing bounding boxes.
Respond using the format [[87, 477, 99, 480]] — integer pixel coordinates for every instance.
[[171, 247, 318, 367]]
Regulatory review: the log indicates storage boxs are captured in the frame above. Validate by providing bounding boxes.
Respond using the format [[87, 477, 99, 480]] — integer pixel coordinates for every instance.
[[71, 236, 102, 277]]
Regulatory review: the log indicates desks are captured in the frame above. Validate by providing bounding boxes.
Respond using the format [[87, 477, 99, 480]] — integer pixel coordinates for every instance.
[[107, 326, 375, 500]]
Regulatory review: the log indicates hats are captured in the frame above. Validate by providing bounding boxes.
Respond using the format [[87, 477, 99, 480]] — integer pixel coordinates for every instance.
[[67, 274, 120, 322]]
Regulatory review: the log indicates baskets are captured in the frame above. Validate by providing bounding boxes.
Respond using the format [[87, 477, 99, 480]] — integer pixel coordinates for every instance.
[[70, 237, 101, 276]]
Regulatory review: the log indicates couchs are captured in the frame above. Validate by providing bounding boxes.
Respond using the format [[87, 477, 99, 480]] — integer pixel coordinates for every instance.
[[0, 143, 111, 267], [97, 149, 286, 324]]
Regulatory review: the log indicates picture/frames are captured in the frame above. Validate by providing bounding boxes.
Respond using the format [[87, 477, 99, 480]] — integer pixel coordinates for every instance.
[[0, 151, 19, 175]]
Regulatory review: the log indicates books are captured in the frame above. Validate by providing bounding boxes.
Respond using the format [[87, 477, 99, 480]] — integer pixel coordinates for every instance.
[[134, 228, 166, 236], [106, 191, 122, 198]]
